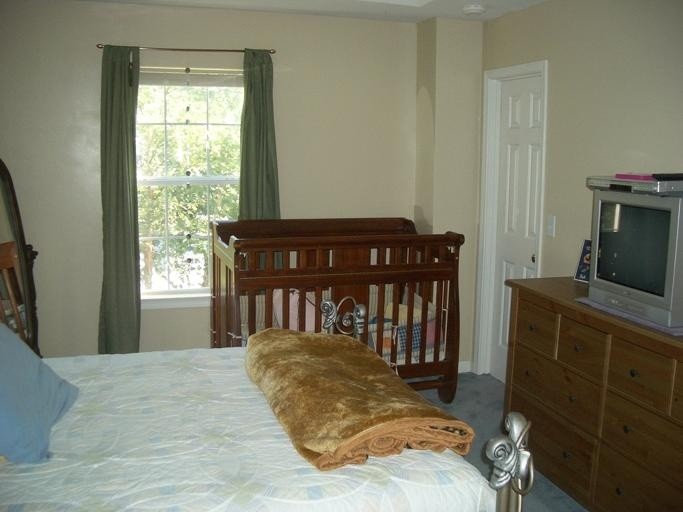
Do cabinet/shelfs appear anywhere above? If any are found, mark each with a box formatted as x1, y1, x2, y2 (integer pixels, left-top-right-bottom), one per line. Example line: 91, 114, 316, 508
503, 276, 683, 512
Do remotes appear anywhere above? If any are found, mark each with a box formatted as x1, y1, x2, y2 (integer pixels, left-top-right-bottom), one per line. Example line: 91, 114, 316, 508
652, 173, 683, 181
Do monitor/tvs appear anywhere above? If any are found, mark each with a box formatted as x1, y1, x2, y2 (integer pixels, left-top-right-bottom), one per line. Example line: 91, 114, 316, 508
588, 189, 683, 328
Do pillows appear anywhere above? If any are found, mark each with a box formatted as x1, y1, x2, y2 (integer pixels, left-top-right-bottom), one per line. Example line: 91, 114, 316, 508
0, 323, 79, 464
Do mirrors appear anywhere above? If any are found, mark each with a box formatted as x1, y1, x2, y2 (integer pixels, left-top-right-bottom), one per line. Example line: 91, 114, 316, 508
0, 158, 43, 358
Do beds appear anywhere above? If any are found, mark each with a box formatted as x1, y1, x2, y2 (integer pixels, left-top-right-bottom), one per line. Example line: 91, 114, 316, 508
208, 217, 464, 404
0, 347, 535, 511
0, 242, 30, 344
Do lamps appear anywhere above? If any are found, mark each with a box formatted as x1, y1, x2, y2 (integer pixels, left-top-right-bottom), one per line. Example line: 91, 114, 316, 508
462, 3, 486, 17
